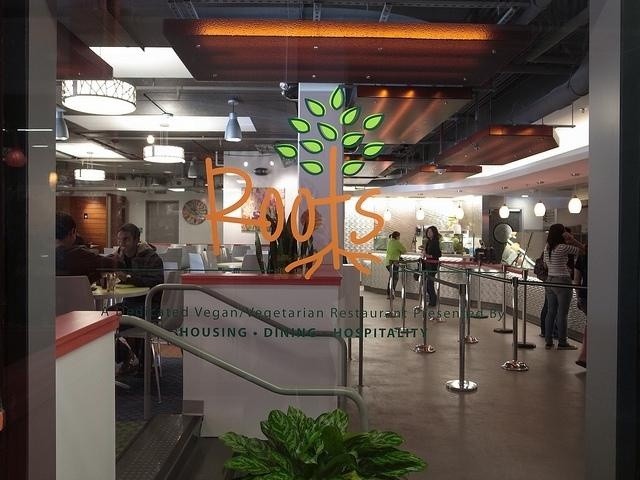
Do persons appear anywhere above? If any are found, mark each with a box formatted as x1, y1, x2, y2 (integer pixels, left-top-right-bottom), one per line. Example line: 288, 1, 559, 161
540, 227, 575, 340
385, 232, 406, 300
542, 223, 585, 350
417, 226, 442, 307
56, 212, 127, 287
106, 222, 164, 380
574, 243, 588, 369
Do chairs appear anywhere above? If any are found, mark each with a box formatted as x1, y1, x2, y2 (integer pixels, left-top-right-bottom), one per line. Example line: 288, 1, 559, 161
55, 243, 280, 404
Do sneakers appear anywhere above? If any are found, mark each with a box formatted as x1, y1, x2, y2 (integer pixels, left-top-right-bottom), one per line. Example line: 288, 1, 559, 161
136, 363, 144, 379
118, 350, 134, 374
545, 343, 553, 349
574, 358, 586, 370
557, 343, 578, 351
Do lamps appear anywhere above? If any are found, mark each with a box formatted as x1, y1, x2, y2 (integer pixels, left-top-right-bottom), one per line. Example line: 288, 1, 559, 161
224, 99, 243, 142
61, 75, 137, 116
142, 131, 186, 164
498, 186, 510, 219
416, 193, 424, 221
455, 189, 464, 219
383, 195, 392, 221
55, 106, 69, 141
74, 159, 106, 182
568, 172, 582, 214
534, 180, 547, 217
187, 161, 198, 178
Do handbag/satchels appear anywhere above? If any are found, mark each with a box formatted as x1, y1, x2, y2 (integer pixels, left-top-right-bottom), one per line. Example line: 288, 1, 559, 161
533, 250, 549, 282
414, 258, 427, 282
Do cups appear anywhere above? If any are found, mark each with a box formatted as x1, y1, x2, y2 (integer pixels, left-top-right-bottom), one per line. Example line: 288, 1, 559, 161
105, 272, 117, 293
